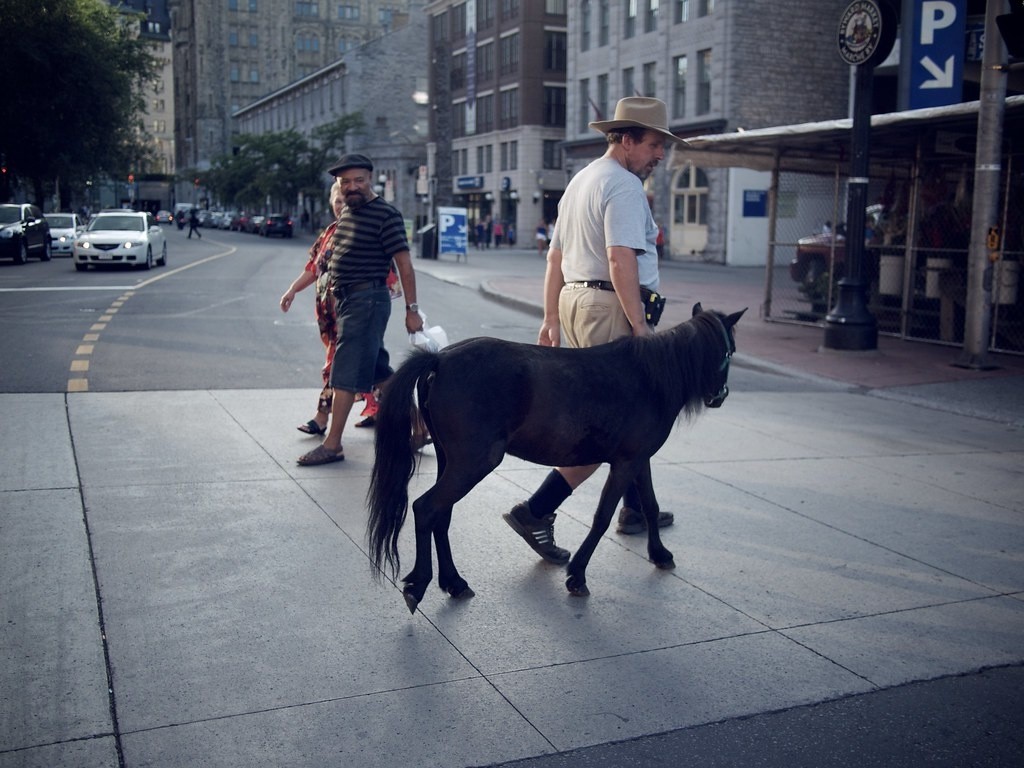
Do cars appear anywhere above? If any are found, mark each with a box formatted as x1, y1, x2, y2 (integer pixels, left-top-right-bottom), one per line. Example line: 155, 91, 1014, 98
41, 212, 87, 257
792, 202, 886, 298
171, 202, 265, 235
73, 209, 167, 271
155, 209, 173, 225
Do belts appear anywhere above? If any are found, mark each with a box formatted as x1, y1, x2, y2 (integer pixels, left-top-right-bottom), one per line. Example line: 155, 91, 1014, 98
579, 280, 652, 305
333, 278, 386, 297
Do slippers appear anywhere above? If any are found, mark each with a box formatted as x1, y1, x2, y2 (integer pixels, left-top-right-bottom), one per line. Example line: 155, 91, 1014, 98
296, 419, 327, 435
296, 443, 345, 466
410, 435, 432, 449
355, 415, 377, 427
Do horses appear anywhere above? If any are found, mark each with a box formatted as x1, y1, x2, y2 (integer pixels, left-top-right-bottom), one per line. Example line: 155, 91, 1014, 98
360, 301, 750, 618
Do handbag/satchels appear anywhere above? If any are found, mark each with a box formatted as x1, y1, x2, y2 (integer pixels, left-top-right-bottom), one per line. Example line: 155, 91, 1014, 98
408, 309, 448, 355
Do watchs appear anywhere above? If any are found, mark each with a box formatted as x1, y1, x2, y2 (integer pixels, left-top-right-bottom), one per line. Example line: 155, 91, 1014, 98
406, 303, 419, 312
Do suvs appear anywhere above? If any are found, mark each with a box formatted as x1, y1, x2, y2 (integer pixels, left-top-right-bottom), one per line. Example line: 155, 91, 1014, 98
0, 202, 53, 266
258, 212, 294, 235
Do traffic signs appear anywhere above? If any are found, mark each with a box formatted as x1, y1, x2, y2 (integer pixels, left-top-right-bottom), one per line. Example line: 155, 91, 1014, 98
437, 211, 469, 255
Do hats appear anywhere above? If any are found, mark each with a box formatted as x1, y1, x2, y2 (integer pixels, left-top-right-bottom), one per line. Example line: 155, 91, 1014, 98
328, 154, 373, 176
589, 97, 691, 146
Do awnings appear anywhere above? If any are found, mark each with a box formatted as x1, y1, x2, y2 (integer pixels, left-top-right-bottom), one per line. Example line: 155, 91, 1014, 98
666, 94, 1024, 318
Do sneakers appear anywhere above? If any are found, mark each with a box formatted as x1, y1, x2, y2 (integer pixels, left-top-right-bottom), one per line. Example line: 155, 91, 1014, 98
616, 508, 674, 534
502, 501, 571, 563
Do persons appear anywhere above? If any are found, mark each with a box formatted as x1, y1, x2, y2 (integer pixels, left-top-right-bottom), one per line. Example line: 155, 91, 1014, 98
279, 153, 433, 465
825, 215, 875, 246
187, 210, 202, 239
657, 223, 667, 257
468, 214, 556, 258
503, 96, 693, 563
